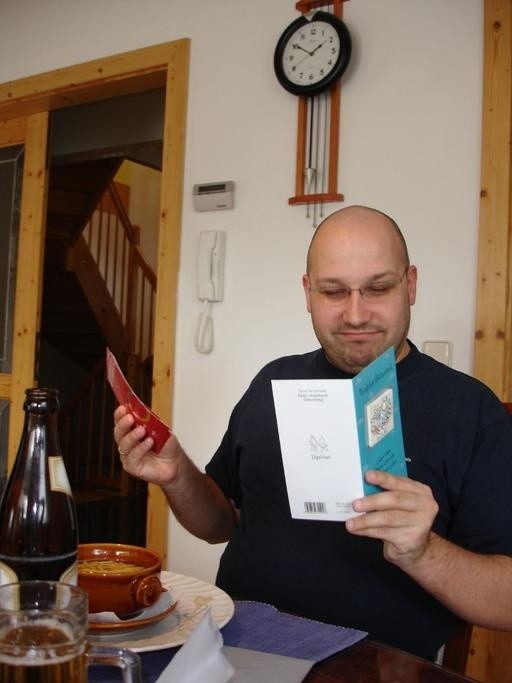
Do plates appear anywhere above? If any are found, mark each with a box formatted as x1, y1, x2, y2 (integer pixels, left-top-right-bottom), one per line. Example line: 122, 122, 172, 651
89, 570, 236, 653
83, 588, 175, 629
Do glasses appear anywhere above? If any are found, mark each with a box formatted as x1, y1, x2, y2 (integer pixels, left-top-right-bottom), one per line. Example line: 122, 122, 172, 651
310, 266, 409, 303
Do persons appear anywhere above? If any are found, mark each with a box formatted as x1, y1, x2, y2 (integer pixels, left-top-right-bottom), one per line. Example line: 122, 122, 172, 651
110, 203, 512, 666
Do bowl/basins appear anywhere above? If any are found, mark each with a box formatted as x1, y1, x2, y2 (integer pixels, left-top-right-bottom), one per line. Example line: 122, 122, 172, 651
78, 544, 161, 615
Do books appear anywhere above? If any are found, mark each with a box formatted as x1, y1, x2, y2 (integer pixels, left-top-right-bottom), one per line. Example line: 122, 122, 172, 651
270, 343, 409, 523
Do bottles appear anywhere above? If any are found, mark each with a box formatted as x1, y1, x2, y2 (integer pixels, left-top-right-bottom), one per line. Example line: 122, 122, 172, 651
0, 388, 78, 625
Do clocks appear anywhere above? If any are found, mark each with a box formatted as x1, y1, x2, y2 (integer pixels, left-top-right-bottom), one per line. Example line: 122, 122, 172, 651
273, 10, 353, 98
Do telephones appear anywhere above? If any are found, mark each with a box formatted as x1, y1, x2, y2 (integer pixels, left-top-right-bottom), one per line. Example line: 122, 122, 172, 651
196, 229, 227, 304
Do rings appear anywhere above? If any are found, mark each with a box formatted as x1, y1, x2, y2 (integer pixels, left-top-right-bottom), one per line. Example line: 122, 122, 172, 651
117, 445, 126, 456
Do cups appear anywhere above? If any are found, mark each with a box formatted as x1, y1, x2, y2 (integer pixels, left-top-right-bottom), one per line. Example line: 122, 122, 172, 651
0, 581, 142, 683
